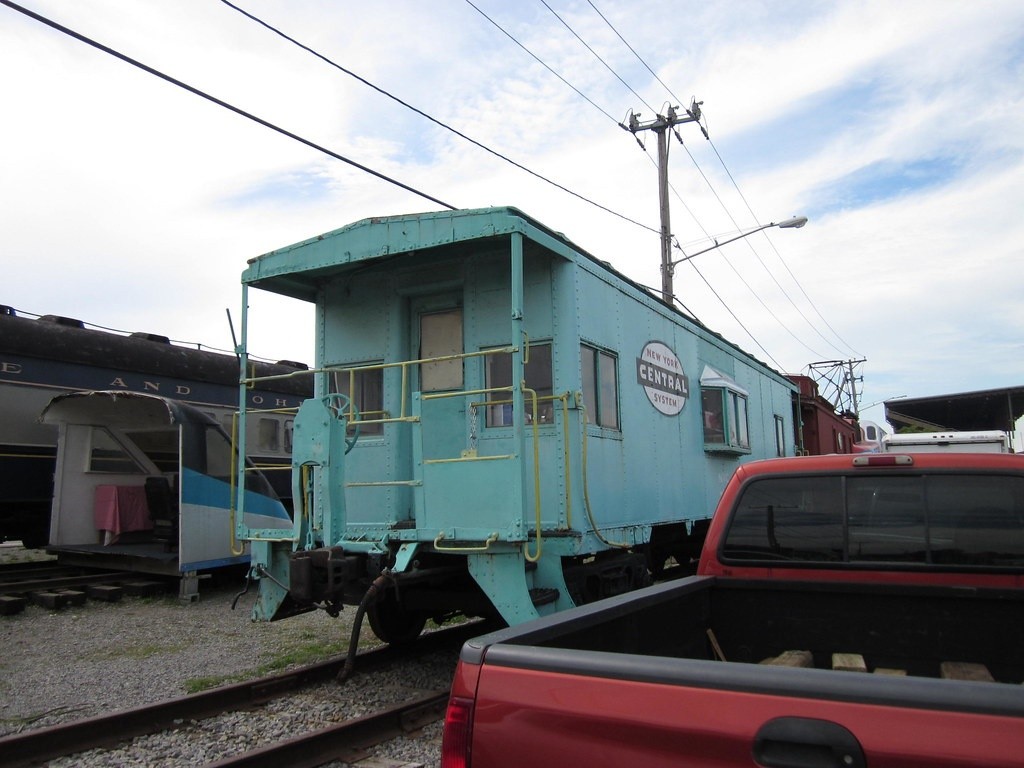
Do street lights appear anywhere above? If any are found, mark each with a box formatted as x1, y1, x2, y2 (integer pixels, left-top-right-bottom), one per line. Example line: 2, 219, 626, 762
662, 215, 808, 307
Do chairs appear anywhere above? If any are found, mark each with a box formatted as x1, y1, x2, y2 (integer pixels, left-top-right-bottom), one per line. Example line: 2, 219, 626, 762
144, 476, 178, 541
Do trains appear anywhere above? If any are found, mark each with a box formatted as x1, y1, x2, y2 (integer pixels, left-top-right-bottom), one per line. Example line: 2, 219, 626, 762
230, 205, 886, 683
1, 301, 314, 550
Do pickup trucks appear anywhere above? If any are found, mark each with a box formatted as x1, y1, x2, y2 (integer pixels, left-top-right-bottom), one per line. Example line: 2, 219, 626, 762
440, 451, 1024, 768
